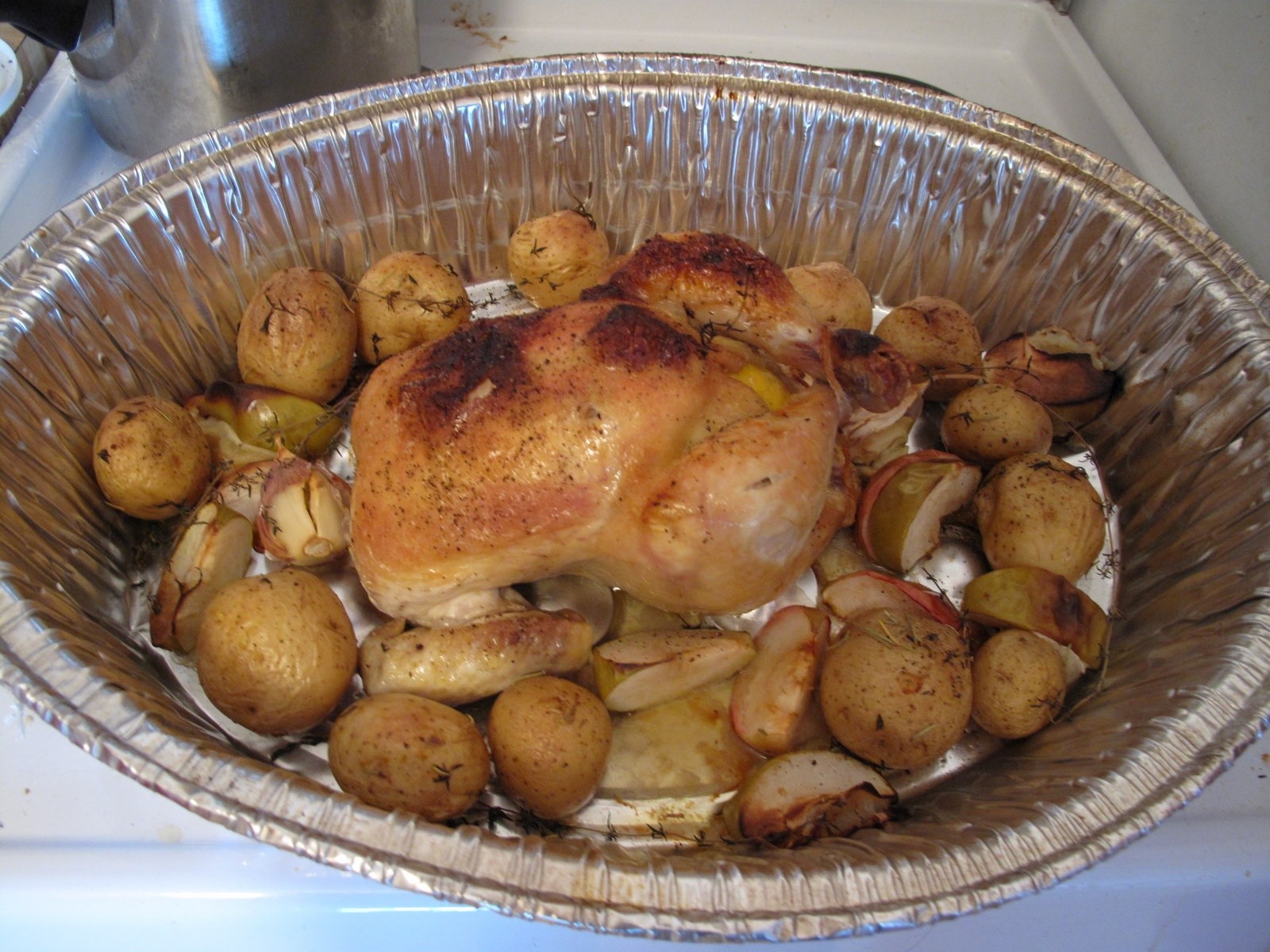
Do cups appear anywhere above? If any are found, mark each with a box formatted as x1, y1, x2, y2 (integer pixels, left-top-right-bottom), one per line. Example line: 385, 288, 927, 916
60, 0, 421, 159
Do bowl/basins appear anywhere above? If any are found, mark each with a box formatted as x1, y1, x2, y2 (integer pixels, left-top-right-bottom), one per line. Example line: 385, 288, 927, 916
0, 51, 1270, 942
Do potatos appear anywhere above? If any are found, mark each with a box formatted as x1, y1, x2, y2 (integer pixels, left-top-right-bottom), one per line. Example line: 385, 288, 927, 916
91, 211, 1107, 823
